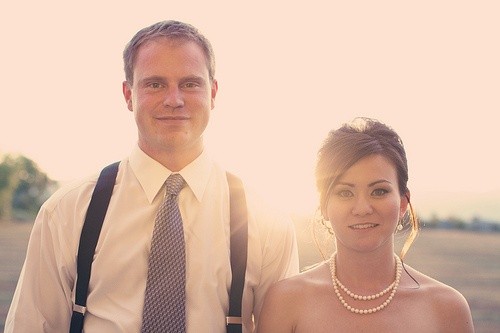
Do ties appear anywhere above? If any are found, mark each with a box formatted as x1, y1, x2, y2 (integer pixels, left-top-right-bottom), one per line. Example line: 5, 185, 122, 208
142, 175, 187, 333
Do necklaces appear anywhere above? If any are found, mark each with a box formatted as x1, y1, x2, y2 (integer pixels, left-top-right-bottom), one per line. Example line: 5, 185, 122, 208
330, 249, 403, 314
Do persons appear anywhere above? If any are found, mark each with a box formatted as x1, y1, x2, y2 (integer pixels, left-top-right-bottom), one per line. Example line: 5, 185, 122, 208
252, 116, 474, 333
4, 20, 301, 333
